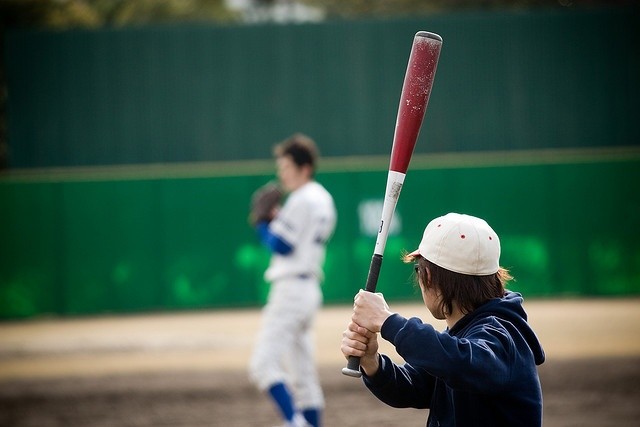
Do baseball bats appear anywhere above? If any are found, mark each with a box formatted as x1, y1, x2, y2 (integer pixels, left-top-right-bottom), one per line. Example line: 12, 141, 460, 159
342, 31, 442, 377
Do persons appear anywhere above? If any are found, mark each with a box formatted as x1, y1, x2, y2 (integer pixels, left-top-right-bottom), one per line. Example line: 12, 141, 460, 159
249, 134, 336, 427
340, 212, 547, 426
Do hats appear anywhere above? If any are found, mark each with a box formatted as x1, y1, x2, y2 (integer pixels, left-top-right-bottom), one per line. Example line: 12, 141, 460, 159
402, 212, 502, 277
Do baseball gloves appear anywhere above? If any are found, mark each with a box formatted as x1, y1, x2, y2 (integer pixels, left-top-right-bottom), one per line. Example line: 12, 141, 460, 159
251, 182, 283, 220
